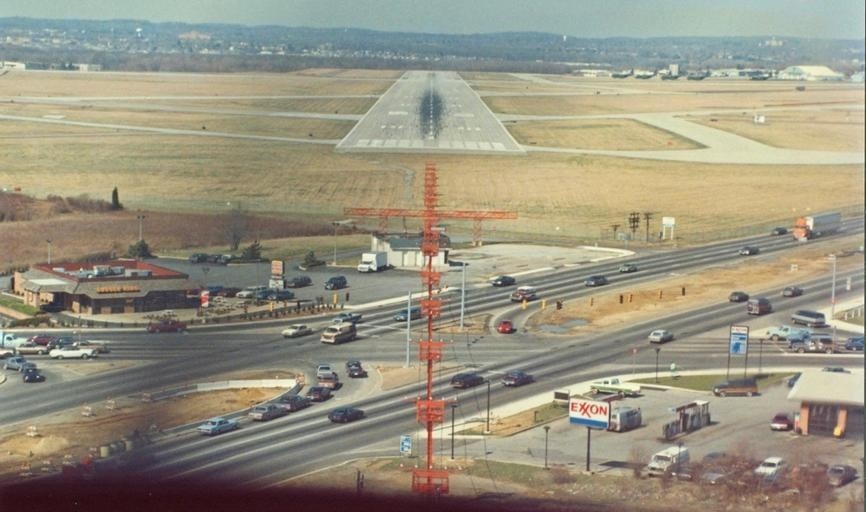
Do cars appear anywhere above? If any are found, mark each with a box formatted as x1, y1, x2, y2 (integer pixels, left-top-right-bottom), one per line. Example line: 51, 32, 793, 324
755, 457, 788, 477
822, 367, 851, 373
283, 323, 312, 338
767, 326, 865, 353
826, 463, 857, 486
741, 246, 760, 256
188, 275, 352, 301
190, 251, 236, 264
148, 320, 186, 334
499, 319, 514, 334
787, 371, 802, 386
772, 227, 788, 235
674, 451, 740, 487
64, 268, 101, 278
770, 412, 792, 431
494, 275, 515, 288
196, 359, 366, 437
504, 370, 535, 385
0, 334, 110, 383
649, 329, 675, 344
620, 263, 639, 272
585, 274, 606, 286
783, 286, 803, 297
730, 290, 749, 304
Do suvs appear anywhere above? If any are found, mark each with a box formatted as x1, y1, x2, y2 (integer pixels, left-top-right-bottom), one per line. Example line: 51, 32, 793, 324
749, 297, 773, 315
451, 372, 484, 388
512, 285, 537, 302
713, 378, 759, 398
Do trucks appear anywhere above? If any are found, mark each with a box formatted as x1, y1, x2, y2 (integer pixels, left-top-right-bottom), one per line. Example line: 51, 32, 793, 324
647, 445, 690, 479
793, 211, 841, 241
356, 251, 388, 274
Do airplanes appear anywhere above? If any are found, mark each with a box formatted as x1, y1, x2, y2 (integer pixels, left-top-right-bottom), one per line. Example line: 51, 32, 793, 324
660, 71, 685, 81
635, 69, 659, 79
752, 72, 774, 80
611, 69, 635, 80
686, 70, 712, 81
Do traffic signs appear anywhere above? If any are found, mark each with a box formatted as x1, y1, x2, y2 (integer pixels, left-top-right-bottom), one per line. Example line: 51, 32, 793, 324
785, 264, 798, 270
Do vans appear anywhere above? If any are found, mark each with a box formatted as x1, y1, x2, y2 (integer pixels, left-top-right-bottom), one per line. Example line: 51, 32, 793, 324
321, 321, 358, 345
610, 405, 643, 432
393, 306, 422, 322
790, 309, 828, 329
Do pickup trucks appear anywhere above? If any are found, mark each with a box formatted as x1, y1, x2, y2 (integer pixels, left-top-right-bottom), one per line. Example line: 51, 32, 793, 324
332, 310, 364, 324
592, 377, 643, 398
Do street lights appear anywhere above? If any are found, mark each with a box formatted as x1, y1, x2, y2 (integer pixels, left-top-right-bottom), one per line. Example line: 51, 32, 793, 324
46, 238, 53, 265
450, 402, 456, 459
759, 337, 765, 372
137, 215, 145, 242
543, 425, 550, 469
654, 347, 660, 384
332, 222, 340, 265
483, 378, 492, 434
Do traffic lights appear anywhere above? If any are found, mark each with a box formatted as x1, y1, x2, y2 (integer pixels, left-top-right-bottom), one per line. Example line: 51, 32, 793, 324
632, 346, 638, 354
